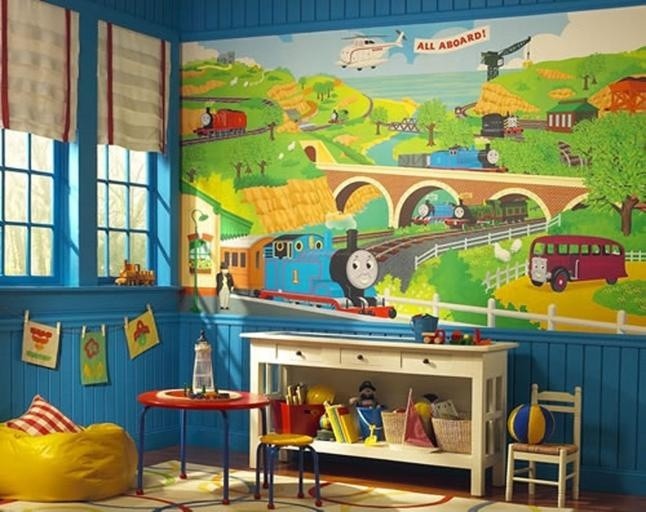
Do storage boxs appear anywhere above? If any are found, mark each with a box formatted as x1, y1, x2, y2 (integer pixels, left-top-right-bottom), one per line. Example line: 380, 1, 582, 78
271, 400, 325, 437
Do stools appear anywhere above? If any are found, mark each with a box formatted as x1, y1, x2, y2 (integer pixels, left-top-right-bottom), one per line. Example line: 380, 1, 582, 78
255, 433, 322, 509
0, 418, 138, 502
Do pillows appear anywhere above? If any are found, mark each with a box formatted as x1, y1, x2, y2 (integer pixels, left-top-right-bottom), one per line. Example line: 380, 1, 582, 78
7, 394, 84, 436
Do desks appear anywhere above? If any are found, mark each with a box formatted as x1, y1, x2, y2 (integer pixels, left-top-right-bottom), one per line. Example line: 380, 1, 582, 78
239, 330, 519, 497
136, 388, 273, 505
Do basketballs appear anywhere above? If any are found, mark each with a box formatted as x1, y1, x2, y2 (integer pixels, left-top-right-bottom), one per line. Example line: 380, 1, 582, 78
507, 404, 554, 444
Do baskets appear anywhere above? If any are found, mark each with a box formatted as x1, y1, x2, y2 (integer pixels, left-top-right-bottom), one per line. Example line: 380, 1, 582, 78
380, 408, 406, 445
431, 411, 472, 454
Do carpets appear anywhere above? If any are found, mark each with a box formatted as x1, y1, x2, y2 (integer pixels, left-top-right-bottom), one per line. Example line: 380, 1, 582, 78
0, 458, 572, 512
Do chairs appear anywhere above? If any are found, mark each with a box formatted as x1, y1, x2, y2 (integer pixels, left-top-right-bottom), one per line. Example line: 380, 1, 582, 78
505, 383, 582, 509
